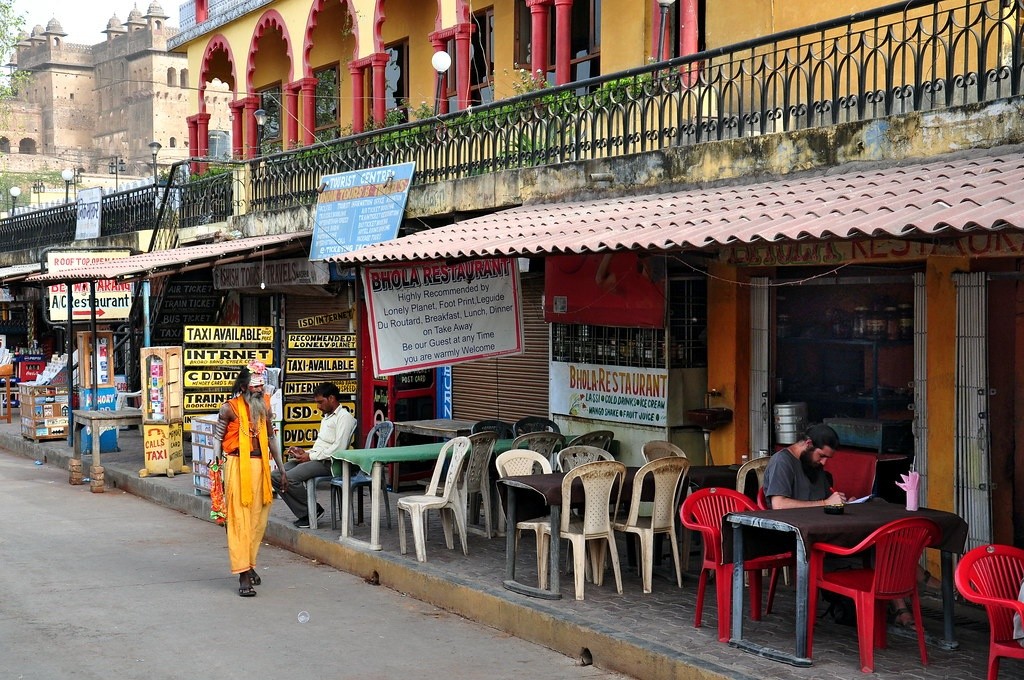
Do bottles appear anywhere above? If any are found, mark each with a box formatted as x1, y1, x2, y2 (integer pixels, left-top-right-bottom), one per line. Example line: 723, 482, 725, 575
741, 455, 747, 464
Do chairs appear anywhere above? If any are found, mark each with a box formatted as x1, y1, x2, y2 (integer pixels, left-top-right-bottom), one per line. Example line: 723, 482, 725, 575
679, 486, 793, 645
757, 486, 797, 616
562, 430, 614, 471
424, 431, 499, 549
396, 435, 471, 563
330, 420, 394, 530
557, 445, 615, 584
510, 431, 567, 474
300, 418, 358, 531
807, 516, 941, 673
471, 420, 514, 439
495, 449, 553, 591
513, 417, 560, 438
736, 456, 792, 588
641, 440, 693, 571
596, 456, 691, 595
540, 460, 628, 601
954, 544, 1024, 680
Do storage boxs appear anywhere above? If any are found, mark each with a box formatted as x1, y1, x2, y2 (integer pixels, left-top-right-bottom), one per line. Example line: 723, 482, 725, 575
17, 361, 45, 382
823, 416, 913, 456
15, 355, 45, 363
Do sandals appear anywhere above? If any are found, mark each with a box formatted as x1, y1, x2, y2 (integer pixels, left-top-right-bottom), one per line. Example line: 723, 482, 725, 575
891, 607, 925, 632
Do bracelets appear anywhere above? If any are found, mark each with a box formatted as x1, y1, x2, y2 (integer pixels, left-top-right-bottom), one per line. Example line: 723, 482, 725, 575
213, 418, 229, 442
268, 434, 276, 440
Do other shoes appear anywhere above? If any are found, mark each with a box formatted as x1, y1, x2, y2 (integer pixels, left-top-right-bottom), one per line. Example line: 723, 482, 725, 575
293, 502, 325, 527
239, 569, 262, 596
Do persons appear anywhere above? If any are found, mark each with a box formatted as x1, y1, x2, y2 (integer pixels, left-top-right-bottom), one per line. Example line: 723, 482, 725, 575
761, 422, 932, 636
269, 381, 358, 529
208, 361, 290, 597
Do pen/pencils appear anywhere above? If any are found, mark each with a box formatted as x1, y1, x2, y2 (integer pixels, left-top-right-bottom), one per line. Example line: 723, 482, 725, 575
285, 447, 302, 451
829, 487, 835, 493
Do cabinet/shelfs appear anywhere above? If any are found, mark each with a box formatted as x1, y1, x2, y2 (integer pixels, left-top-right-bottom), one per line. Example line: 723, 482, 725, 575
76, 330, 115, 389
140, 346, 184, 425
0, 364, 14, 424
775, 337, 913, 421
17, 381, 69, 444
190, 414, 218, 498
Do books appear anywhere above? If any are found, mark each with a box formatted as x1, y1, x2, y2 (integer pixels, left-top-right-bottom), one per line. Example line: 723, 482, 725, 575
79, 336, 109, 387
147, 356, 181, 422
18, 352, 73, 437
191, 413, 223, 491
0, 348, 12, 367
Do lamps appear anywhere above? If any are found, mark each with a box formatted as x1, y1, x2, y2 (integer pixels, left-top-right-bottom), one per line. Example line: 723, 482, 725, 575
108, 155, 126, 193
149, 142, 162, 183
62, 170, 74, 203
9, 186, 21, 216
657, 0, 675, 77
253, 109, 269, 158
431, 51, 451, 152
33, 179, 46, 210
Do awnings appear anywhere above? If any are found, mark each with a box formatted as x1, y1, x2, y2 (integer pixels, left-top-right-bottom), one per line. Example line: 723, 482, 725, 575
318, 146, 1024, 267
0, 228, 313, 288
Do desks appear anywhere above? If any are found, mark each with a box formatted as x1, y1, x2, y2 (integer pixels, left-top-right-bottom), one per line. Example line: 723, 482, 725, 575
393, 418, 480, 493
330, 438, 529, 553
721, 502, 970, 669
69, 409, 143, 466
495, 464, 759, 601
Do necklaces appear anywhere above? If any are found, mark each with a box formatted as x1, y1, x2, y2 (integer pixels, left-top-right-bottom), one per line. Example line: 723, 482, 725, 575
238, 398, 265, 438
